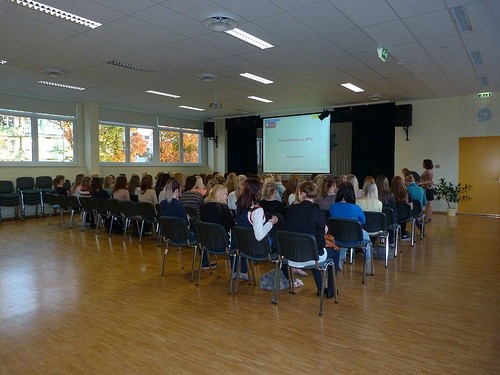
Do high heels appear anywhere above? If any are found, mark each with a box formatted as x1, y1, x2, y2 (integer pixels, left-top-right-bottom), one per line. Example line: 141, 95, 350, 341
425, 219, 432, 223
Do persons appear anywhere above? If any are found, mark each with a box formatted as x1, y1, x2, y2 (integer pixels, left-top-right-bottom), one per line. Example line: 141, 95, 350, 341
420, 159, 436, 223
284, 182, 339, 299
330, 182, 374, 275
53, 168, 427, 254
235, 178, 301, 288
199, 184, 253, 281
158, 179, 216, 269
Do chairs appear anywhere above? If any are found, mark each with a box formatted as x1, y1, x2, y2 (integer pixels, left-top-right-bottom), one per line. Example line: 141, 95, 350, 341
0, 176, 427, 316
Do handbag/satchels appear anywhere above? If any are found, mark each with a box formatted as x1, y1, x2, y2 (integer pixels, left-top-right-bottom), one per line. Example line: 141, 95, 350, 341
104, 218, 123, 234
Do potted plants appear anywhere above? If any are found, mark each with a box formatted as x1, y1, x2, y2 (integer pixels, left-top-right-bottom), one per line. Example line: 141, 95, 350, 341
433, 178, 474, 216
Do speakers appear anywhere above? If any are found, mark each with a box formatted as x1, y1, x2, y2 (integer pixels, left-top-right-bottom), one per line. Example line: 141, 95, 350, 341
395, 103, 412, 126
203, 122, 214, 138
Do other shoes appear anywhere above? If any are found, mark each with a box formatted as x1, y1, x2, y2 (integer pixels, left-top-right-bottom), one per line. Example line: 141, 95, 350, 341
202, 263, 217, 269
233, 272, 253, 280
318, 287, 327, 295
401, 235, 411, 240
86, 221, 91, 225
91, 224, 99, 229
326, 289, 338, 298
